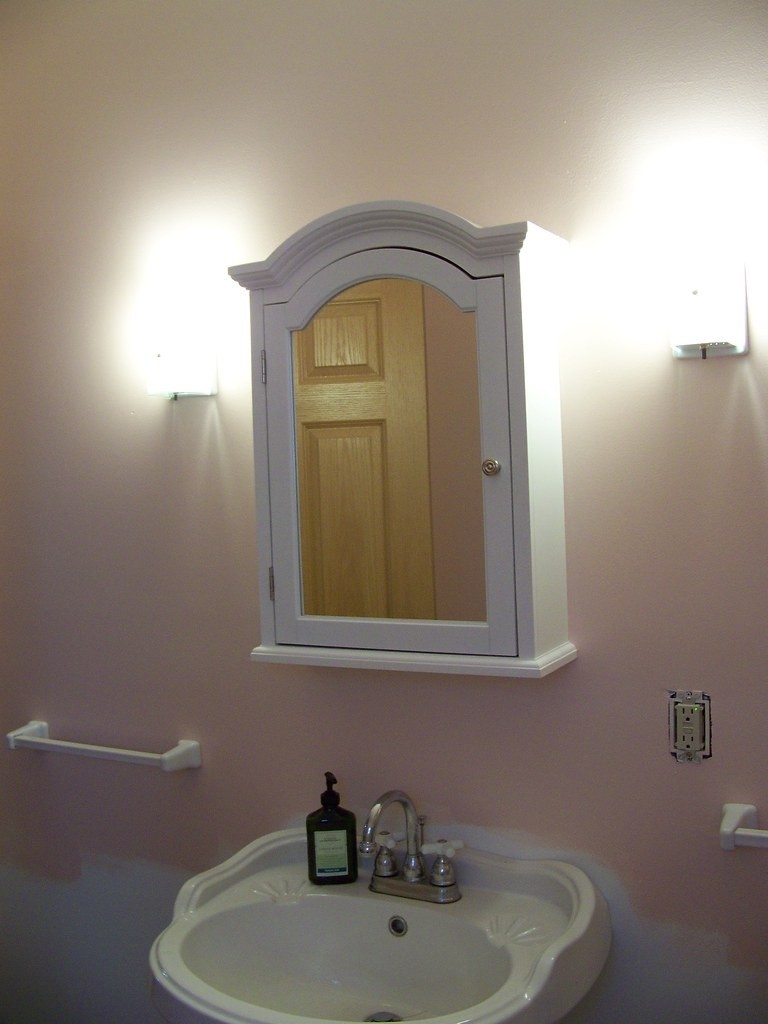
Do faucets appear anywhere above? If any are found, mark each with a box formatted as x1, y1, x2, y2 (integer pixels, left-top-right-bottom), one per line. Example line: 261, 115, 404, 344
358, 789, 427, 882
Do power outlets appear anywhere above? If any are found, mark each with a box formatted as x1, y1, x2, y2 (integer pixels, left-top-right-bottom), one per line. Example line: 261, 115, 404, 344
669, 691, 710, 764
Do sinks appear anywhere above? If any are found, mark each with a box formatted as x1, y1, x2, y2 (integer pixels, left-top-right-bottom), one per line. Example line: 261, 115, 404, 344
147, 823, 611, 1024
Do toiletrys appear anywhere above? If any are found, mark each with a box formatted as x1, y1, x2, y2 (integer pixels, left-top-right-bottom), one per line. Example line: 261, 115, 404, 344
305, 771, 357, 886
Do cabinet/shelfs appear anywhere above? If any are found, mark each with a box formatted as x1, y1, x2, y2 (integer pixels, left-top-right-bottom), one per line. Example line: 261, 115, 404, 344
229, 200, 576, 677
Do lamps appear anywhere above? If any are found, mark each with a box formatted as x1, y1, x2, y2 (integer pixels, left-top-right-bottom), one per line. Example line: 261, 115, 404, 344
667, 253, 749, 359
170, 334, 216, 399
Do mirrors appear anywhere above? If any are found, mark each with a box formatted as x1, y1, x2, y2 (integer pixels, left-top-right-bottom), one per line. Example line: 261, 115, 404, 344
264, 249, 517, 657
